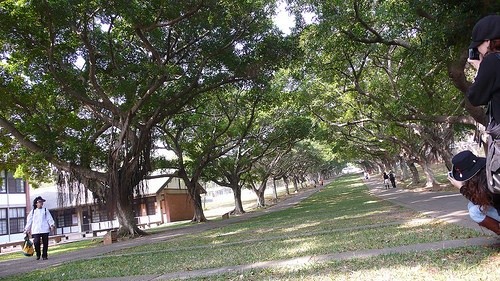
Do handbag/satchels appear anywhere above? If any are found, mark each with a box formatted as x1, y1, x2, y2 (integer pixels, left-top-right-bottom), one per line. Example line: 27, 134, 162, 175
485, 120, 500, 194
22, 232, 34, 256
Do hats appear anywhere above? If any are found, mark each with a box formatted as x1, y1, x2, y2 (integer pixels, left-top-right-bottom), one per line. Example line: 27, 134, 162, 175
448, 150, 486, 182
34, 197, 46, 203
467, 15, 500, 49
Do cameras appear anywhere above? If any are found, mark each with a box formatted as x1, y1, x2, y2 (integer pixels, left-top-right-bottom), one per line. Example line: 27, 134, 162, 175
449, 172, 453, 177
469, 49, 480, 60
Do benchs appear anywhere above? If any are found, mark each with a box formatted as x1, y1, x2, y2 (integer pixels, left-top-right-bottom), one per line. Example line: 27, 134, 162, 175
90, 221, 163, 236
0, 234, 66, 253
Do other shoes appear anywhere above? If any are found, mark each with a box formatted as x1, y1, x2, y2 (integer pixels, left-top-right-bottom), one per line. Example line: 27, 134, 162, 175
43, 257, 48, 260
36, 256, 40, 260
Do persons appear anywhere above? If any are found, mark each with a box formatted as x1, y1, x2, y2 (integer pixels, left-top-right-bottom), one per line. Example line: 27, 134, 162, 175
467, 15, 500, 193
383, 172, 390, 190
447, 150, 500, 235
24, 196, 55, 260
389, 172, 396, 188
